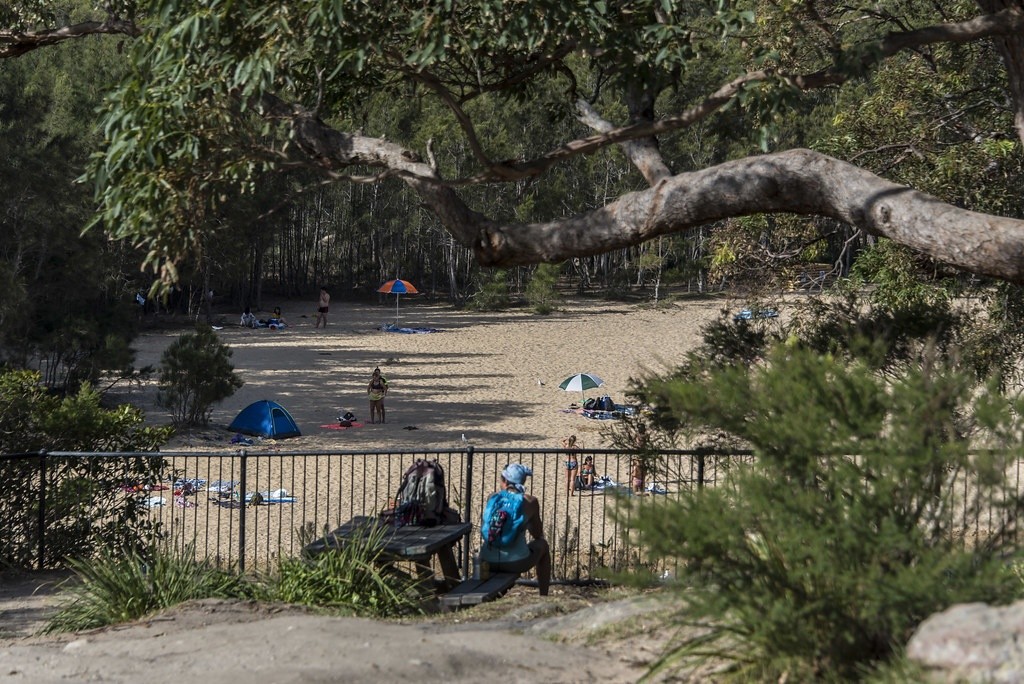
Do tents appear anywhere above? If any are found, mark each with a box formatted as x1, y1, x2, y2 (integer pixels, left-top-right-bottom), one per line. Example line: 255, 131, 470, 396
227, 398, 302, 440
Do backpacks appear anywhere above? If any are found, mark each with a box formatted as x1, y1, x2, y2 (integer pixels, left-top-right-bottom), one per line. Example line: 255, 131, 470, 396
394, 458, 447, 528
480, 489, 526, 547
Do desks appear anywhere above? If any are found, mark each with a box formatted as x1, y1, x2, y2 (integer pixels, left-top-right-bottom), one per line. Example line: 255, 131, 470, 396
300, 515, 472, 602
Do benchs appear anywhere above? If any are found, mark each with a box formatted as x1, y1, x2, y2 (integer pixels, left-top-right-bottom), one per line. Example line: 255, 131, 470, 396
732, 308, 780, 325
441, 573, 521, 612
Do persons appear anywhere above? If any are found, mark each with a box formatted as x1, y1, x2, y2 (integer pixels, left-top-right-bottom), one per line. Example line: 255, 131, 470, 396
314, 286, 330, 329
562, 434, 582, 497
241, 306, 257, 329
367, 365, 389, 424
478, 463, 551, 597
577, 455, 599, 490
272, 306, 293, 328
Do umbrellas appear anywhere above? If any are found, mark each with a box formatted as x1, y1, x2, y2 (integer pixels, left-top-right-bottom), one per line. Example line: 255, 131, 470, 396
736, 306, 778, 332
559, 372, 604, 406
376, 275, 420, 329
